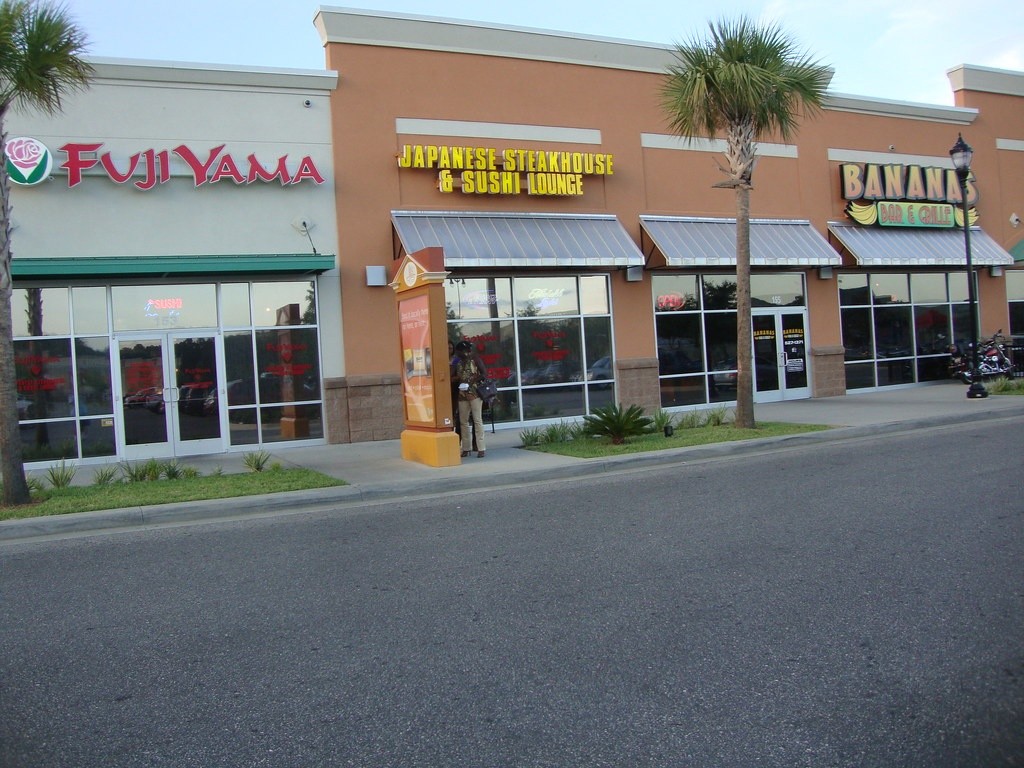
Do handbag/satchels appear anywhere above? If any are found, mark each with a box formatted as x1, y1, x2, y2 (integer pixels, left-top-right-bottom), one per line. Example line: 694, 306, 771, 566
476, 381, 498, 400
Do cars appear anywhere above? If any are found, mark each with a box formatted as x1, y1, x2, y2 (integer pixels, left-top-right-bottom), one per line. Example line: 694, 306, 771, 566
179, 373, 310, 417
843, 335, 944, 361
16, 390, 52, 419
497, 362, 568, 389
710, 357, 791, 391
569, 357, 614, 389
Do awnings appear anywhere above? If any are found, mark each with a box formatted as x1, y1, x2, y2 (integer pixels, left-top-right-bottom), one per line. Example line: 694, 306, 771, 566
639, 214, 843, 267
390, 210, 645, 266
826, 221, 1015, 266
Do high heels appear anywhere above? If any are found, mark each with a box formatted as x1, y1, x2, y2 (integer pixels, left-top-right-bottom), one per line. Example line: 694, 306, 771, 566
461, 451, 471, 456
478, 451, 485, 458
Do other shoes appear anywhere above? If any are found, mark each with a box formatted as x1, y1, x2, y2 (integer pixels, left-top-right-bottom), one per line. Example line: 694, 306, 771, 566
473, 442, 478, 451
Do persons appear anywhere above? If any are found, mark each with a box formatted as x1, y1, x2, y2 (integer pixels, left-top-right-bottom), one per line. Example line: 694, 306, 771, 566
68, 383, 90, 443
448, 339, 487, 458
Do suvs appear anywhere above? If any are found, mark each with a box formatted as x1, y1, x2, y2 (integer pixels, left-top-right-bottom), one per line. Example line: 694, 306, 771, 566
124, 386, 165, 413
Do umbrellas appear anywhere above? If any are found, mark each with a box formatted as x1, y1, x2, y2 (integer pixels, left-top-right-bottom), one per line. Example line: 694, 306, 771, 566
914, 310, 957, 350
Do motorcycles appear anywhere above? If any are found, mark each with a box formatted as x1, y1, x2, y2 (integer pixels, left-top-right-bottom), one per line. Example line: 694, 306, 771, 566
948, 328, 1015, 385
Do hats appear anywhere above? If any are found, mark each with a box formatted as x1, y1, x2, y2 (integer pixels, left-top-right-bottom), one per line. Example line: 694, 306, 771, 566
455, 341, 472, 351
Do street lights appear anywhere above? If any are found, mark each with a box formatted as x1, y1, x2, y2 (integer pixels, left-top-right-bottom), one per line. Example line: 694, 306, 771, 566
950, 131, 993, 400
